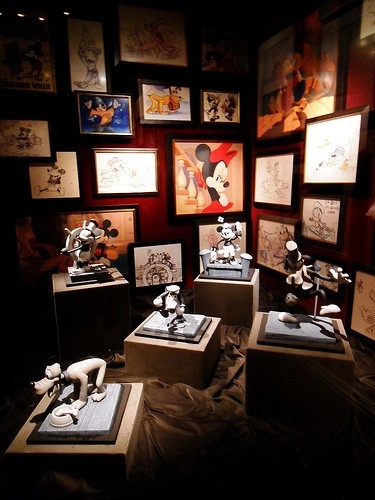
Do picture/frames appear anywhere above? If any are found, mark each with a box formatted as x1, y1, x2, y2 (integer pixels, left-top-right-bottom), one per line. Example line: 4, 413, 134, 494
309, 254, 349, 303
345, 261, 375, 352
128, 238, 186, 293
137, 78, 194, 124
192, 220, 251, 277
167, 133, 249, 226
299, 193, 345, 250
52, 205, 141, 269
0, 0, 161, 204
199, 88, 242, 126
253, 16, 371, 210
254, 215, 301, 278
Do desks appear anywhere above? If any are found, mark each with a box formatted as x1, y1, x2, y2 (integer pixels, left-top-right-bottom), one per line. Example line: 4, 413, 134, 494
243, 311, 355, 415
6, 382, 146, 500
124, 310, 225, 389
